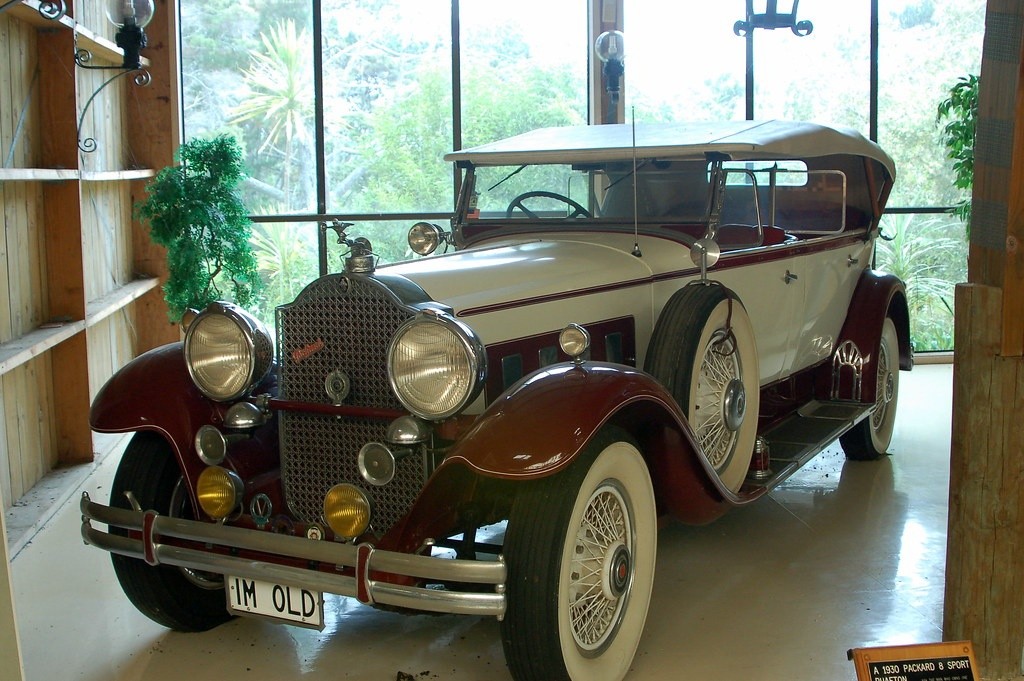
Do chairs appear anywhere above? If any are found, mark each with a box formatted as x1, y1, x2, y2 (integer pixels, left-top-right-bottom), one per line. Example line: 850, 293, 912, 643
766, 197, 870, 233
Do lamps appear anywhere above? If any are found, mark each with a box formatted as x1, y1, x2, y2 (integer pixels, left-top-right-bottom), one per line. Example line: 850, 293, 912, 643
73, 0, 156, 154
595, 30, 627, 125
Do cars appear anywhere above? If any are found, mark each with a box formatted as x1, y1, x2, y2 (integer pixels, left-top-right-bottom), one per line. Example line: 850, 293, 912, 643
79, 105, 914, 681
